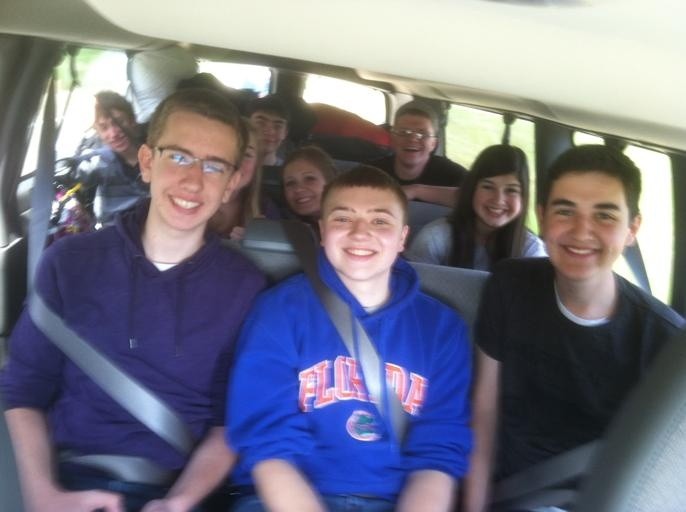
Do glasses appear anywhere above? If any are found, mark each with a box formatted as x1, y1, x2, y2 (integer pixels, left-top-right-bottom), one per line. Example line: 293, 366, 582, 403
150, 146, 238, 180
389, 127, 438, 142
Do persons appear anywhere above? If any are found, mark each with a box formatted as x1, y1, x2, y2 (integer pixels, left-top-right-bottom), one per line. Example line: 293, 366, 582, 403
0, 68, 686, 512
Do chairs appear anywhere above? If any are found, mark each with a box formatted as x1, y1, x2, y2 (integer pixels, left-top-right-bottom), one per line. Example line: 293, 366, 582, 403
545, 328, 686, 512
